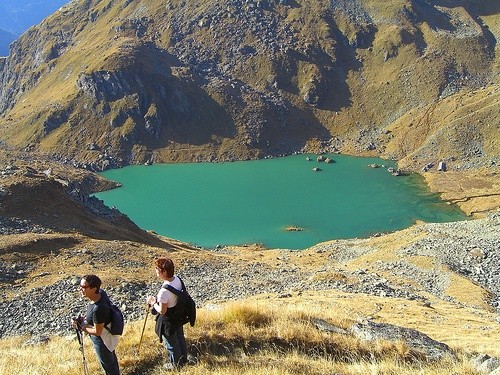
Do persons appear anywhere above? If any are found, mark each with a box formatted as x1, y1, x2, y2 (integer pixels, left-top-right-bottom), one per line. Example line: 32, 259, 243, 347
72, 274, 120, 375
147, 258, 187, 371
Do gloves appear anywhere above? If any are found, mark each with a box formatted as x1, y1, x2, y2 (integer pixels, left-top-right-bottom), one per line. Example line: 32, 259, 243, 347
147, 295, 156, 305
72, 313, 86, 331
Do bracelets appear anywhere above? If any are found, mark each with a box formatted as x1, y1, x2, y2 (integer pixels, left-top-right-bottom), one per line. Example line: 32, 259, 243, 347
153, 302, 156, 308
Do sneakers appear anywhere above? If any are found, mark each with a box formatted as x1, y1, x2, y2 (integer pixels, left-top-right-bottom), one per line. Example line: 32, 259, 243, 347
163, 363, 175, 371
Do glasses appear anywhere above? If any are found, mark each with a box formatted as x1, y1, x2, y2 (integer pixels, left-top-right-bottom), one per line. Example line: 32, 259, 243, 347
79, 284, 90, 289
156, 266, 162, 269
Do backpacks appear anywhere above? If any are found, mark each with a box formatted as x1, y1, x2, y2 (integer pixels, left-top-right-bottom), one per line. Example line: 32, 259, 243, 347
163, 276, 196, 327
92, 289, 124, 335
